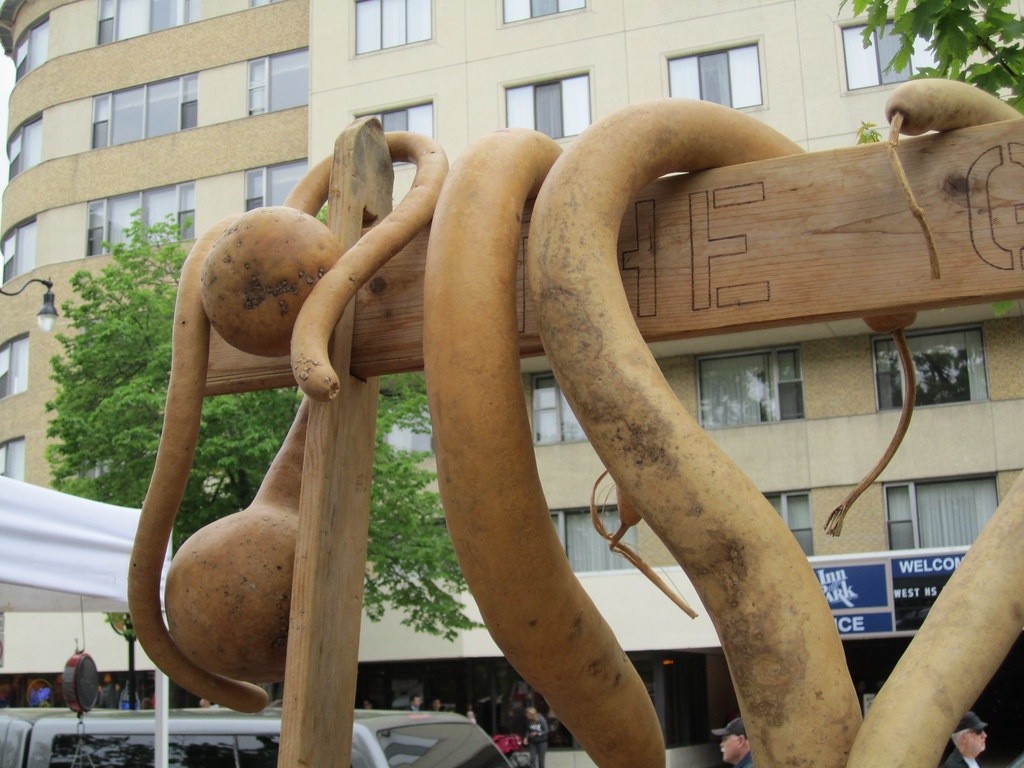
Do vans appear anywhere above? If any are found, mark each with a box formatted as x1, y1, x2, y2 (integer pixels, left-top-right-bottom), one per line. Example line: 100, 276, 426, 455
0, 696, 514, 768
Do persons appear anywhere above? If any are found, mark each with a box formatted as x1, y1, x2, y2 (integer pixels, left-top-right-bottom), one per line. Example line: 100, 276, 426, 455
200, 695, 1024, 768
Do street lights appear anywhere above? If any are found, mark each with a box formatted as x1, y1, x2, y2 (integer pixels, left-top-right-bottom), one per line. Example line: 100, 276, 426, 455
106, 609, 139, 711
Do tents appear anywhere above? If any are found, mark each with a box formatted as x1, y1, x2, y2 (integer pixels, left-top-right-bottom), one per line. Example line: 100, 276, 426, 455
0, 478, 172, 768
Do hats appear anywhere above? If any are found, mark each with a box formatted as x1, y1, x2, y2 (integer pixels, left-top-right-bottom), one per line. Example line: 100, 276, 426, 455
711, 717, 745, 736
954, 711, 988, 733
